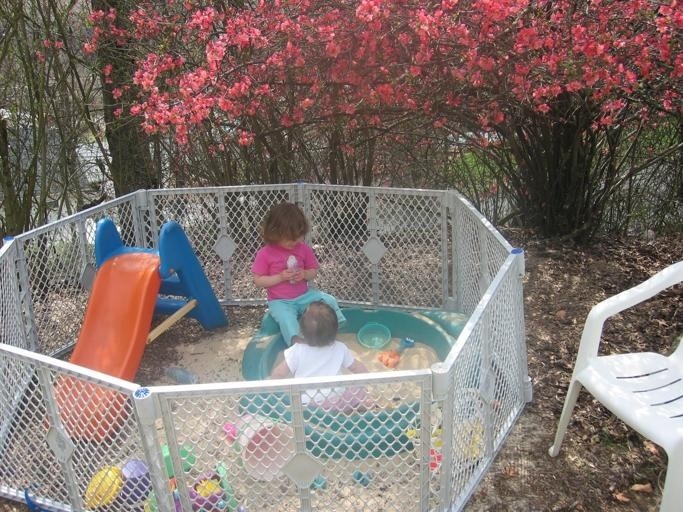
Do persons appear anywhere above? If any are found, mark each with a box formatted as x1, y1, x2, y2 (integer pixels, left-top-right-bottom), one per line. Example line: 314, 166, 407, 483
251, 202, 346, 348
265, 301, 377, 415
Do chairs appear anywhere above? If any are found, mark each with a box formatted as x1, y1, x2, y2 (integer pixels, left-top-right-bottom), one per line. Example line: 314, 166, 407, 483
548, 260, 683, 512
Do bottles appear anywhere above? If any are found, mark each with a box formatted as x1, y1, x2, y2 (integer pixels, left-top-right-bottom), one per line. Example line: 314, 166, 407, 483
167, 368, 199, 384
287, 255, 298, 282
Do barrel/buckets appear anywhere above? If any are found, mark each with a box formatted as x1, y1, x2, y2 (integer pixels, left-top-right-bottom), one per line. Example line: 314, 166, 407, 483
236, 415, 295, 482
